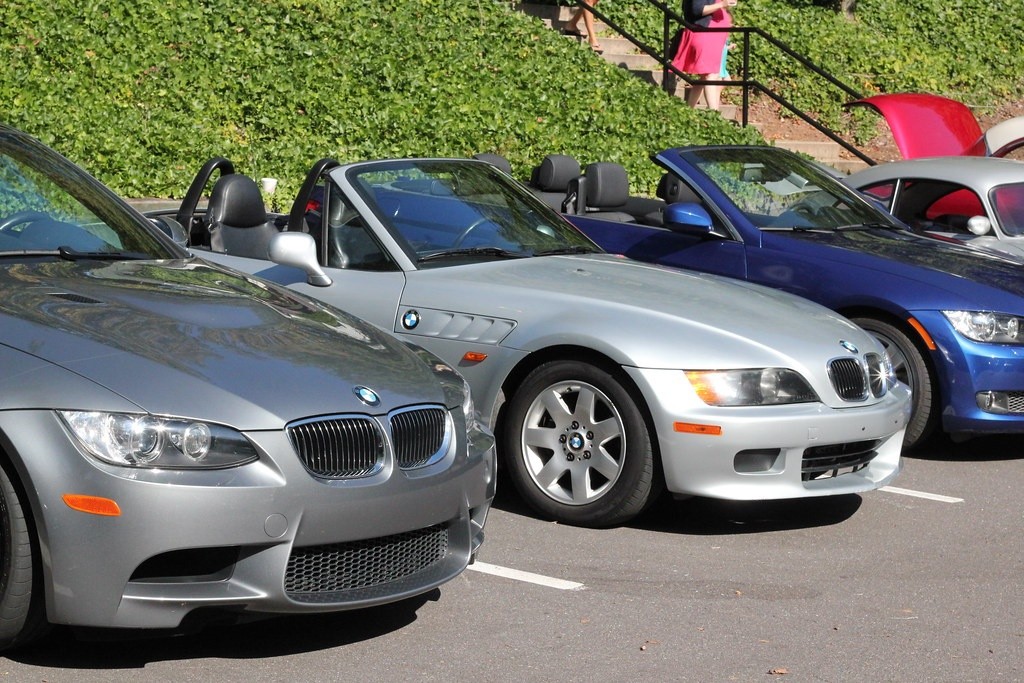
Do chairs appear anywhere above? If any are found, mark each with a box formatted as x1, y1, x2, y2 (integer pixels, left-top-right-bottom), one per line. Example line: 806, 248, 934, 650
666, 169, 729, 236
204, 174, 281, 261
330, 176, 388, 269
585, 161, 637, 226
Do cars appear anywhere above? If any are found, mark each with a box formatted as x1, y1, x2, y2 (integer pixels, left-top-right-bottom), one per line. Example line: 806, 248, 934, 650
844, 93, 1024, 226
784, 154, 1023, 265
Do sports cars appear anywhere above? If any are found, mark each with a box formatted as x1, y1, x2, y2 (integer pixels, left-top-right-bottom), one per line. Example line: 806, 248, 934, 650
0, 120, 499, 640
71, 157, 912, 529
367, 141, 1024, 448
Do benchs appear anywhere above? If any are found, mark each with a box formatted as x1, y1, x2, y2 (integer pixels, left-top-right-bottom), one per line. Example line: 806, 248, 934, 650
466, 154, 581, 213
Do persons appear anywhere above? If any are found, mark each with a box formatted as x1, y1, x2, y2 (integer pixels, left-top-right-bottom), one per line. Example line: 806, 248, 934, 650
668, 0, 737, 113
565, 0, 604, 55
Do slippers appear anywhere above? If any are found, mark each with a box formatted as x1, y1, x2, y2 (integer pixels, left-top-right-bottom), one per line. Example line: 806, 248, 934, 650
560, 27, 588, 38
587, 43, 606, 54
